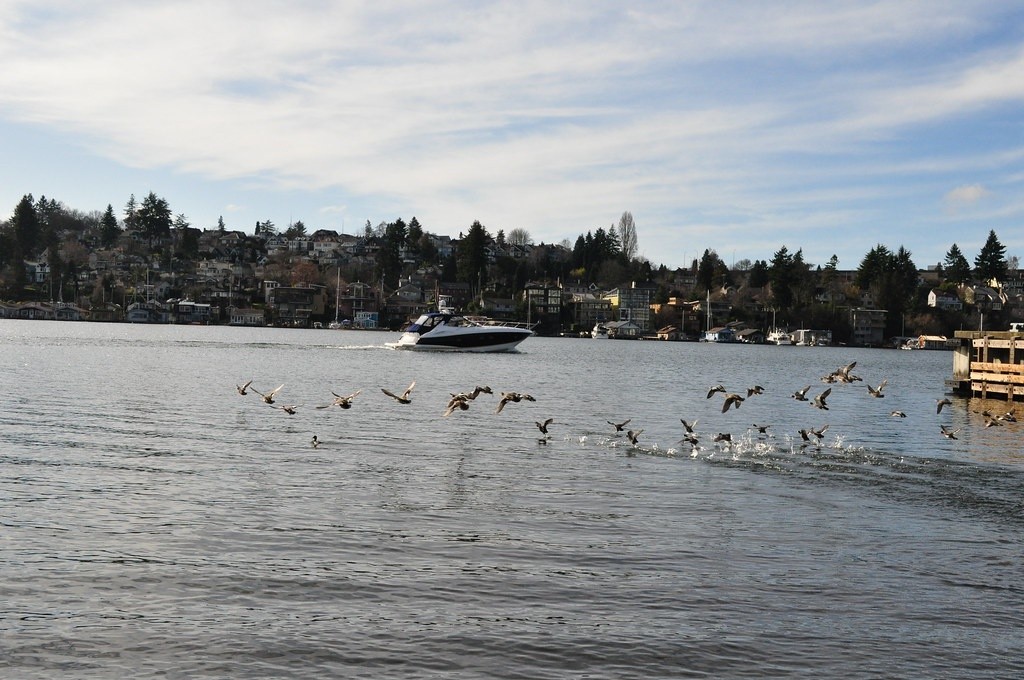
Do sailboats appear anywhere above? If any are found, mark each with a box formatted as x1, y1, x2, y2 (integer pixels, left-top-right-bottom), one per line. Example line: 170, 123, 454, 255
328, 267, 345, 330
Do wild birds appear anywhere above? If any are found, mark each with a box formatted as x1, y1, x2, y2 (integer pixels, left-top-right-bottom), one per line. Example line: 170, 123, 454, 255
235, 359, 1019, 446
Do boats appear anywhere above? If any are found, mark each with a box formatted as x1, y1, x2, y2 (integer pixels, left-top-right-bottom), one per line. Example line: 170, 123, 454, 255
384, 294, 542, 353
767, 310, 793, 345
